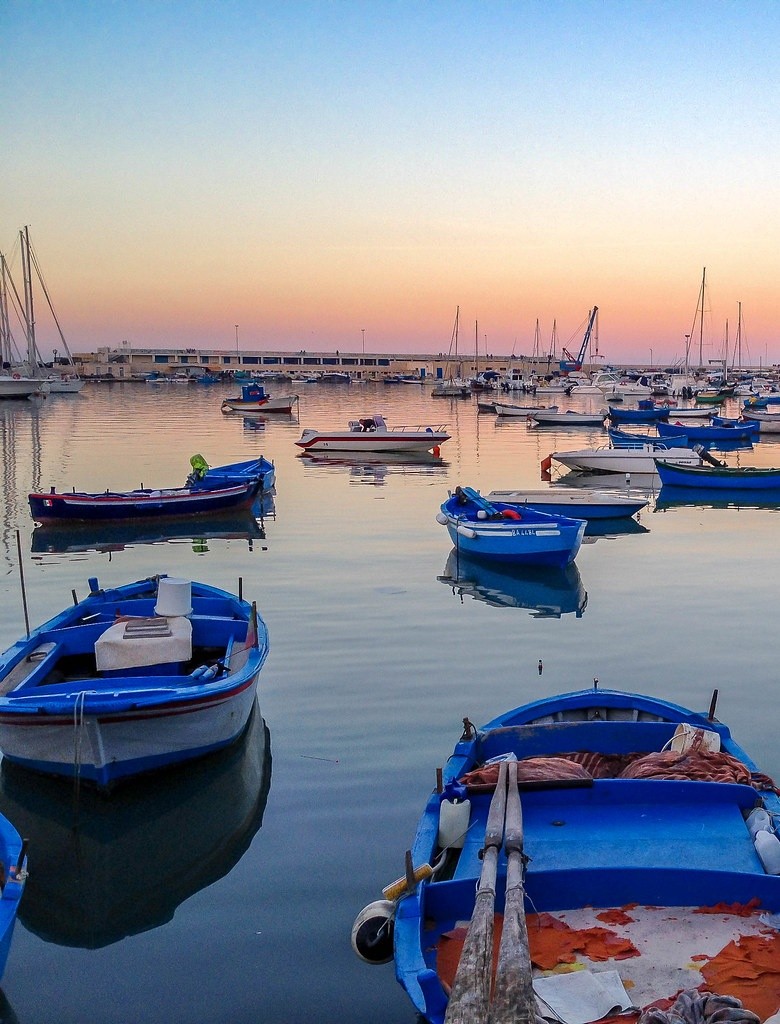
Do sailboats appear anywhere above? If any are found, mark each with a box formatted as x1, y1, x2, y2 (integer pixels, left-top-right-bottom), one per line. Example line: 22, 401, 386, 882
0, 226, 85, 398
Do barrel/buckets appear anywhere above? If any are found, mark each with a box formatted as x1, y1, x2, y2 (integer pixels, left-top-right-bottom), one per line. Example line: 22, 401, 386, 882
671, 723, 721, 754
154, 578, 193, 616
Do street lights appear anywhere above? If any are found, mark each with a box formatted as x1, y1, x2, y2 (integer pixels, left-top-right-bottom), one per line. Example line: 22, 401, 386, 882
360, 328, 365, 353
235, 324, 239, 364
685, 334, 690, 348
649, 348, 652, 364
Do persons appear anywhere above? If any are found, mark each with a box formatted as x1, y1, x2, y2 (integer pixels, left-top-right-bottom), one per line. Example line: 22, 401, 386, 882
360, 419, 377, 432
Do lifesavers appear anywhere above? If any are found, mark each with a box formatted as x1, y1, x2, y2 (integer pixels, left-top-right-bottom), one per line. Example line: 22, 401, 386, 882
501, 509, 523, 520
12, 372, 21, 379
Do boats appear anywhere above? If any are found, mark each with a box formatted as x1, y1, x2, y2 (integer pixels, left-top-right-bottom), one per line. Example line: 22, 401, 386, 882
351, 379, 367, 383
539, 442, 729, 475
293, 414, 453, 452
220, 382, 298, 413
347, 680, 780, 1024
29, 509, 266, 554
221, 409, 297, 430
1, 572, 269, 791
27, 472, 265, 526
293, 450, 443, 466
144, 368, 352, 384
248, 486, 277, 520
652, 483, 780, 513
653, 456, 780, 491
382, 266, 780, 448
183, 454, 276, 492
484, 489, 650, 519
436, 545, 588, 619
0, 694, 274, 950
435, 486, 588, 568
580, 518, 650, 544
370, 378, 379, 382
541, 469, 663, 490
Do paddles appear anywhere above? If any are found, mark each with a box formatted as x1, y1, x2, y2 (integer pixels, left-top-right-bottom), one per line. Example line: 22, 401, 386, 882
490, 761, 543, 1024
463, 485, 505, 519
443, 757, 507, 1024
